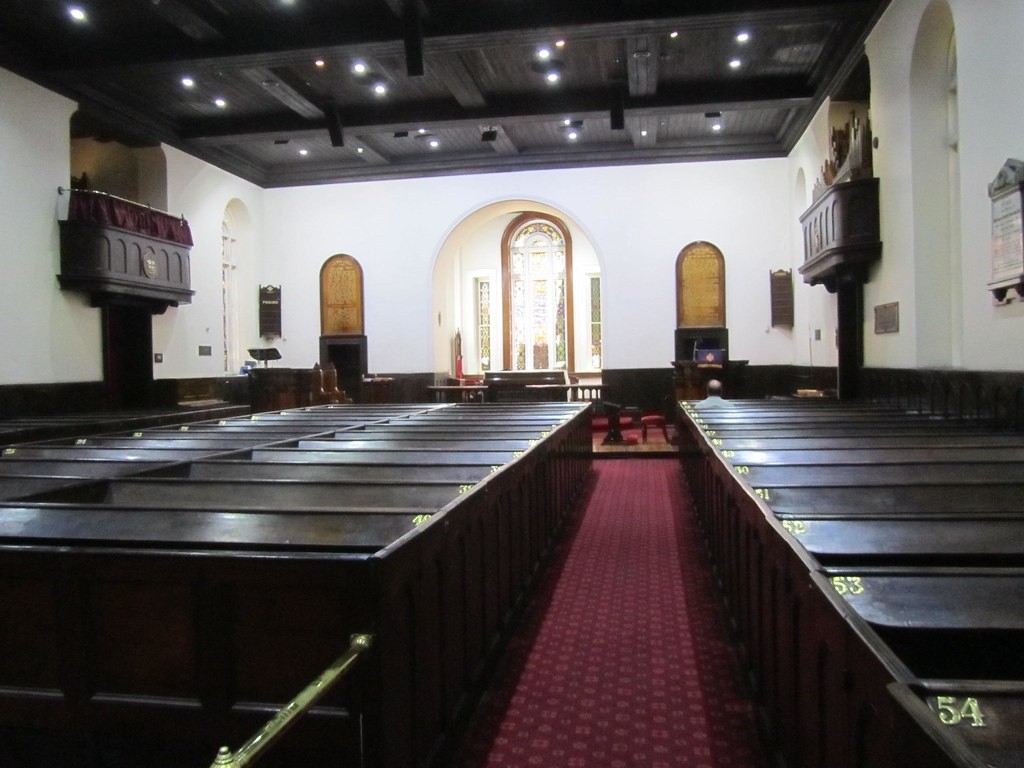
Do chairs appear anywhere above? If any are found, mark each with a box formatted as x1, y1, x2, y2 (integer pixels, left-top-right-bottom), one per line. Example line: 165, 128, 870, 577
312, 363, 335, 406
322, 362, 353, 403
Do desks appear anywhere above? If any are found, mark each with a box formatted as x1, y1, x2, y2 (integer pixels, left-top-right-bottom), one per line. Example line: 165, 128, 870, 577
482, 372, 568, 402
676, 398, 1023, 768
0, 403, 593, 768
361, 377, 396, 404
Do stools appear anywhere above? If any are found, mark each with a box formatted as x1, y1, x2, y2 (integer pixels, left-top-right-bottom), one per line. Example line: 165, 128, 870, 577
641, 415, 670, 444
